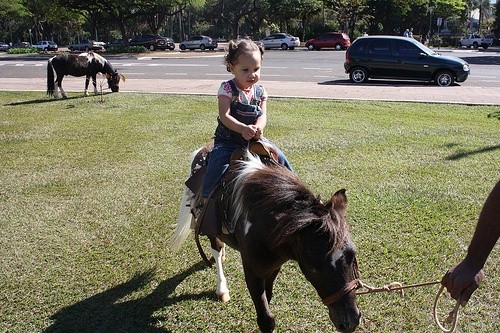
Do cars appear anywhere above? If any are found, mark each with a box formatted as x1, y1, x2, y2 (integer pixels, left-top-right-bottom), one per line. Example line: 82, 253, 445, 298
0, 42, 9, 52
343, 36, 470, 87
179, 36, 218, 51
305, 32, 350, 51
256, 33, 300, 51
68, 34, 175, 53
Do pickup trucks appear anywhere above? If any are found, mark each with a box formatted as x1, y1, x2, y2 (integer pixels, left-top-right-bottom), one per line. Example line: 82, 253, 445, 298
458, 34, 493, 50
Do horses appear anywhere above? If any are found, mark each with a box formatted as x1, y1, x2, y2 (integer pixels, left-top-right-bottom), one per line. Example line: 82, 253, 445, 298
45, 51, 122, 101
164, 141, 365, 333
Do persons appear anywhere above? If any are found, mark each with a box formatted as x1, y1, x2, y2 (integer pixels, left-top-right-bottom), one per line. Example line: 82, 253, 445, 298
441, 181, 500, 308
193, 40, 296, 220
404, 28, 432, 48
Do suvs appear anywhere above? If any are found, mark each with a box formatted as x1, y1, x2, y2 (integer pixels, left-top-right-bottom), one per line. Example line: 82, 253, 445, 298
30, 41, 58, 51
